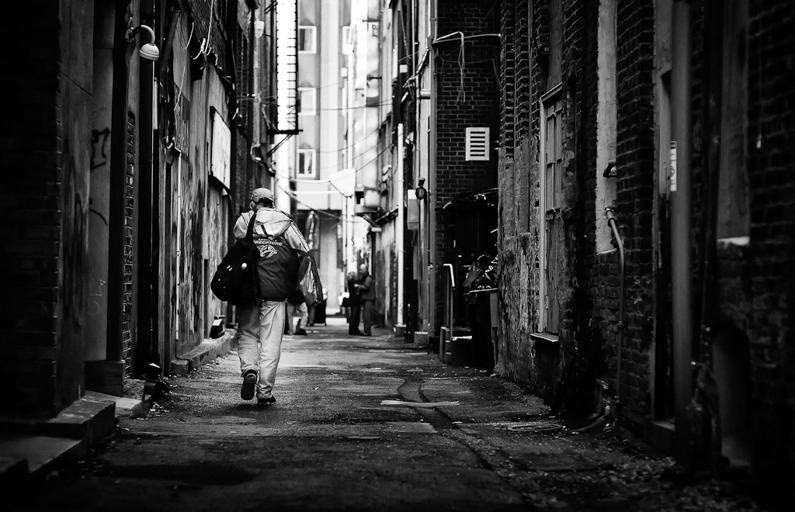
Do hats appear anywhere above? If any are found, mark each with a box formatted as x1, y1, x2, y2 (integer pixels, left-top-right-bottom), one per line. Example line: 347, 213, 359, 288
251, 188, 274, 202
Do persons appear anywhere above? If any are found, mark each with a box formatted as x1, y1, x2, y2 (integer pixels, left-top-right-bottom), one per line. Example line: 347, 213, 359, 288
307, 288, 317, 326
346, 271, 363, 336
286, 289, 308, 336
237, 189, 310, 405
354, 264, 375, 336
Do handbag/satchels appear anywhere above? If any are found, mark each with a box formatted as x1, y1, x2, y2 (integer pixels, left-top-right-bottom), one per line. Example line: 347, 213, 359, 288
258, 234, 309, 301
210, 239, 262, 308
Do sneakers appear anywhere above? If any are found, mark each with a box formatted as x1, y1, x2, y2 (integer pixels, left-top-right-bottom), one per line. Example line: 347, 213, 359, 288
241, 369, 276, 405
